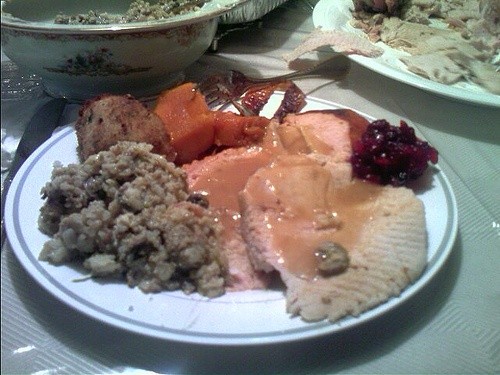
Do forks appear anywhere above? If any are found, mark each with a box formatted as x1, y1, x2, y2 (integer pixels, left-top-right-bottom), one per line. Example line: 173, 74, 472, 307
194, 54, 352, 110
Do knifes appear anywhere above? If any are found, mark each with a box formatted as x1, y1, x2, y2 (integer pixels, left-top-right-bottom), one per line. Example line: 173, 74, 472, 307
0, 95, 67, 261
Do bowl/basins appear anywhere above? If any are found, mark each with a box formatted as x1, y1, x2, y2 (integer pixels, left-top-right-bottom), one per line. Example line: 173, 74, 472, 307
0, 0, 253, 102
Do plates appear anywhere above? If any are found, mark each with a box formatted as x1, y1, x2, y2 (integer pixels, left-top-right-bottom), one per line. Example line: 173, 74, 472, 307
4, 86, 461, 347
311, 0, 500, 110
